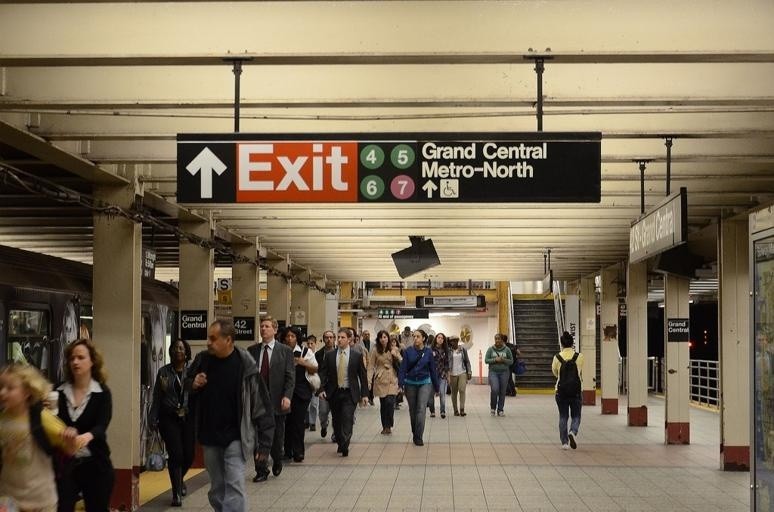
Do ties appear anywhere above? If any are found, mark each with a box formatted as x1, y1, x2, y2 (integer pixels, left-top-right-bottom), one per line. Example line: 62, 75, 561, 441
337, 352, 344, 386
260, 345, 269, 387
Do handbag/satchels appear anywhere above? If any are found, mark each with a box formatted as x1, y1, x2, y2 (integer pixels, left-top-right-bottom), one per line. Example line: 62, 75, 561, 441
146, 431, 165, 471
305, 368, 321, 391
513, 359, 526, 374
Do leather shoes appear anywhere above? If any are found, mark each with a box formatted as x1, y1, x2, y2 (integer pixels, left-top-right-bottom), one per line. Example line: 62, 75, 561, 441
272, 462, 282, 477
172, 479, 187, 507
253, 472, 268, 482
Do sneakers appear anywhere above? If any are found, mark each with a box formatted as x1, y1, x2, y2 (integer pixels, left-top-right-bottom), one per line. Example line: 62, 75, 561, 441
441, 413, 446, 418
561, 432, 578, 450
430, 413, 435, 417
285, 448, 304, 462
490, 409, 504, 417
454, 408, 466, 416
321, 428, 327, 437
414, 437, 423, 445
380, 428, 391, 434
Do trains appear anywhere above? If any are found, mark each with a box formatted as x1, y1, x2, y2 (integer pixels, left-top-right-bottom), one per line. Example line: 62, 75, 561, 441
0, 244, 178, 472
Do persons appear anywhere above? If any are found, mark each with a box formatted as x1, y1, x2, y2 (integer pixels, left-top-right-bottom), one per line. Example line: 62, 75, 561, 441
42, 336, 114, 512
551, 329, 585, 451
752, 325, 773, 478
279, 325, 369, 463
485, 334, 520, 417
248, 315, 296, 482
366, 326, 472, 434
0, 359, 83, 512
398, 330, 438, 446
146, 338, 197, 508
181, 318, 275, 512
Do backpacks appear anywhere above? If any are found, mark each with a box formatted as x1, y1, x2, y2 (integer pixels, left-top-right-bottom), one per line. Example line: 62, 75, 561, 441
555, 352, 580, 396
